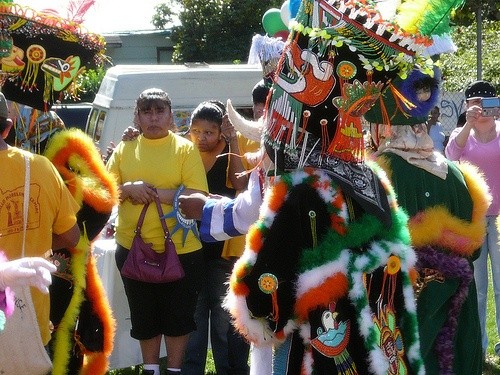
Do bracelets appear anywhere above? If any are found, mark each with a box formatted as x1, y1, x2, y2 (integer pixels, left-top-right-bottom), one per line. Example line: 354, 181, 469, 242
225, 136, 237, 142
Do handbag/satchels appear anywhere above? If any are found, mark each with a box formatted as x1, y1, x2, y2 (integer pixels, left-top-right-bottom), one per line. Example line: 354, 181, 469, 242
0, 286, 53, 375
121, 188, 184, 282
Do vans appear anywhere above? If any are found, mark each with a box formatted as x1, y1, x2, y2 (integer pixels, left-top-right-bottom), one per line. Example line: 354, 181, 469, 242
85, 62, 266, 164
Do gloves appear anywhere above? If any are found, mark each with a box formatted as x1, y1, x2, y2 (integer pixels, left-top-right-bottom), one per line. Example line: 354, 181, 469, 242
0, 256, 57, 294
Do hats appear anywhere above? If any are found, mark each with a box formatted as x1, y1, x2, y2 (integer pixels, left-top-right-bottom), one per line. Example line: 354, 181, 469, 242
464, 81, 497, 100
259, 0, 431, 174
0, 92, 9, 119
361, 0, 468, 128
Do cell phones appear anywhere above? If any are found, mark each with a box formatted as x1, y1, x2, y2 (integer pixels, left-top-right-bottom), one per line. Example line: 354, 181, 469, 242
480, 97, 500, 117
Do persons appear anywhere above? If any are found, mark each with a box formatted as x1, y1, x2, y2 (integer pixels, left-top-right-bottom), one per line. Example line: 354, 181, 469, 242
0, 0, 492, 375
445, 81, 500, 370
